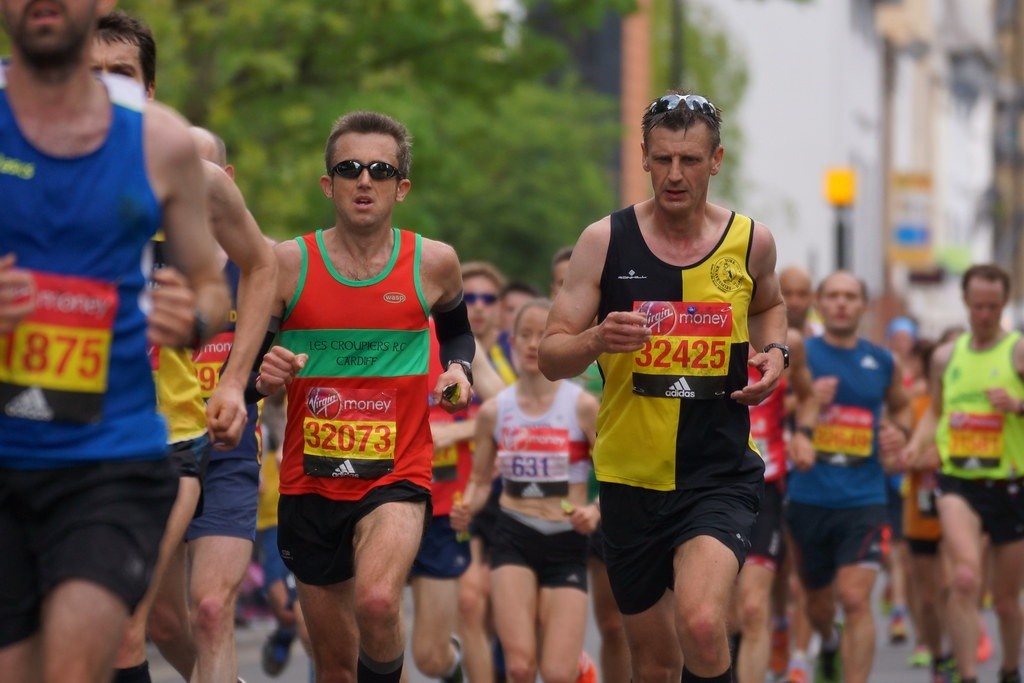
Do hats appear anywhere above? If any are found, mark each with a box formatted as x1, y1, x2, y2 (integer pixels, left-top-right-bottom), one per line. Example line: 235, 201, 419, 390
886, 314, 914, 338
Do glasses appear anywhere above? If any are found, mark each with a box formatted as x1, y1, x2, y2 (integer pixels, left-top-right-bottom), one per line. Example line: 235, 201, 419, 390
463, 293, 497, 306
330, 160, 403, 180
648, 94, 716, 115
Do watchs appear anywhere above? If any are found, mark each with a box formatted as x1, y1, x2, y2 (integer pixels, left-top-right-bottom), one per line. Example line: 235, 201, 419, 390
763, 342, 790, 369
194, 311, 206, 350
446, 358, 473, 386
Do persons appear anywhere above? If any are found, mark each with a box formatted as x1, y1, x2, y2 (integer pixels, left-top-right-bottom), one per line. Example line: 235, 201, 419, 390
747, 265, 1024, 683
90, 13, 317, 683
538, 95, 788, 683
219, 112, 476, 683
406, 246, 634, 682
0, 0, 231, 683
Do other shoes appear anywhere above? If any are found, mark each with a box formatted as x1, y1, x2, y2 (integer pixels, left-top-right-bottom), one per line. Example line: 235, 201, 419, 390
909, 637, 933, 667
441, 635, 465, 683
262, 618, 296, 676
785, 656, 812, 683
578, 651, 597, 683
976, 632, 992, 662
889, 610, 906, 644
930, 655, 960, 683
813, 623, 845, 683
770, 623, 789, 675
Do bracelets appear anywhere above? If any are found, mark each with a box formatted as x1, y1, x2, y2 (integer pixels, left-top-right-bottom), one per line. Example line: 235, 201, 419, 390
796, 425, 813, 437
